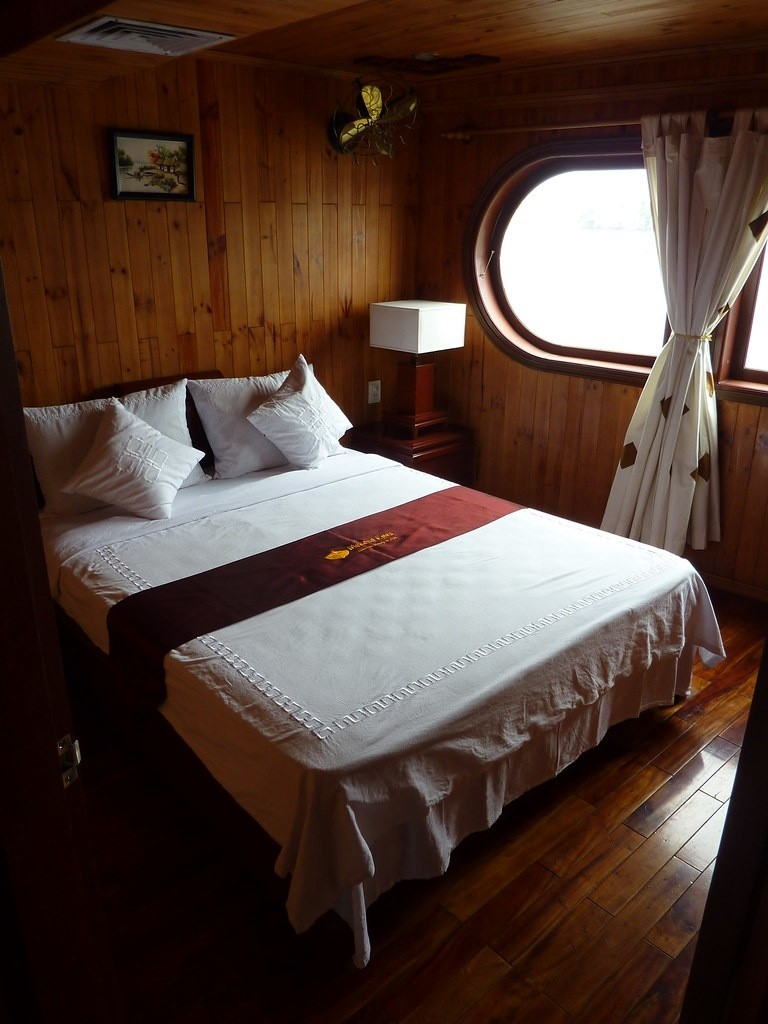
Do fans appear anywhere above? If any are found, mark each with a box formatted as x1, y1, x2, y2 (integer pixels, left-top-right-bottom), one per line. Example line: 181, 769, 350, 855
327, 70, 417, 168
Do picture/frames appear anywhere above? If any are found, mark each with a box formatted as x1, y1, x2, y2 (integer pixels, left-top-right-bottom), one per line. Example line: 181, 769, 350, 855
106, 128, 196, 202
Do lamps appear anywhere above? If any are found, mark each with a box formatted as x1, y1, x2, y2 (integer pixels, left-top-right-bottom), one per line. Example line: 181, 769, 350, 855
369, 299, 467, 439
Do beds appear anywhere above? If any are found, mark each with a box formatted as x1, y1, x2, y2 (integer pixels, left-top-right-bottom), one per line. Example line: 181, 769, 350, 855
22, 369, 726, 970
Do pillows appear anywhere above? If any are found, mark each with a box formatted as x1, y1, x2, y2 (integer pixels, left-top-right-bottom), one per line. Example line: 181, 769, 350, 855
186, 364, 321, 480
59, 396, 206, 520
23, 378, 212, 519
247, 353, 354, 467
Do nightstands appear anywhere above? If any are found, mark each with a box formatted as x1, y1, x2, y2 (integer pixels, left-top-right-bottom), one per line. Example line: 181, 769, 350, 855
352, 425, 473, 480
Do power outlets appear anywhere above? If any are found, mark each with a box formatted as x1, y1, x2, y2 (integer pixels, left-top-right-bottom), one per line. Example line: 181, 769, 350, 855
367, 380, 381, 404
